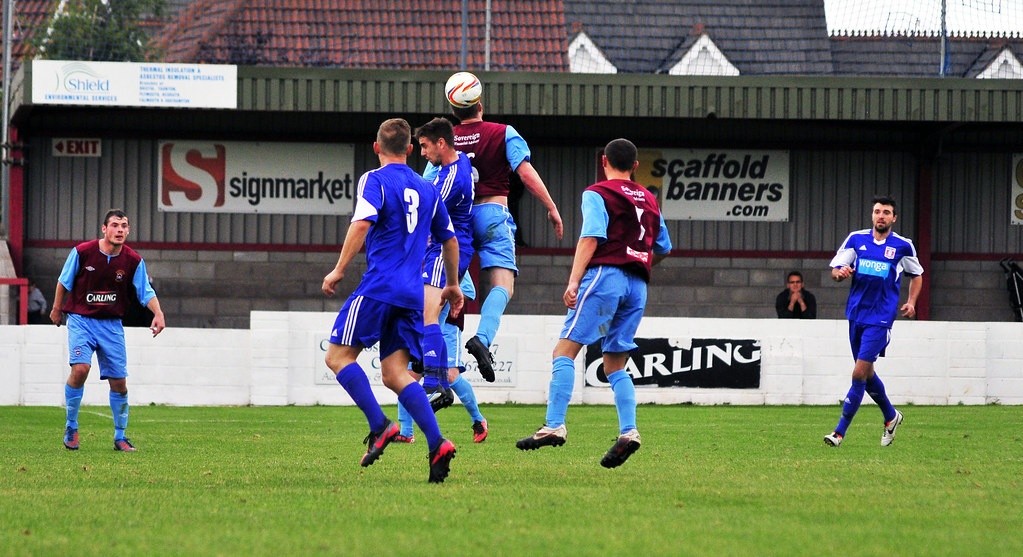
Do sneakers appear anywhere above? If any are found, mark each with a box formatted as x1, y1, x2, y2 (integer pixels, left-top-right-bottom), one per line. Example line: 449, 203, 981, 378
114, 440, 138, 453
360, 418, 400, 467
465, 336, 495, 382
424, 383, 454, 413
64, 426, 79, 450
880, 409, 903, 447
516, 424, 567, 450
396, 435, 415, 443
600, 429, 641, 469
428, 436, 456, 483
824, 432, 843, 447
472, 419, 488, 443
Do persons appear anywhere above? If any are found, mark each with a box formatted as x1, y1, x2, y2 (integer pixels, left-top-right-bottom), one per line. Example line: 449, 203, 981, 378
28, 280, 48, 325
823, 197, 924, 447
776, 271, 817, 319
422, 100, 563, 383
49, 210, 166, 452
402, 117, 475, 413
321, 118, 464, 483
516, 138, 672, 469
387, 269, 488, 442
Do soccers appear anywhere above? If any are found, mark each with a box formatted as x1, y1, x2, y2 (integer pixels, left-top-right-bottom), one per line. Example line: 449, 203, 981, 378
444, 71, 483, 109
